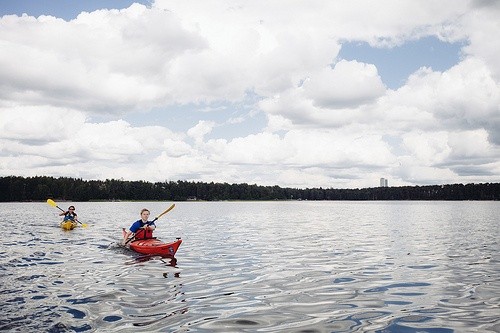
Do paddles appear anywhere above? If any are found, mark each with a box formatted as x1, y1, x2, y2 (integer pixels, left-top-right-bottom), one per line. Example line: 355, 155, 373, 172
125, 203, 175, 244
47, 199, 88, 228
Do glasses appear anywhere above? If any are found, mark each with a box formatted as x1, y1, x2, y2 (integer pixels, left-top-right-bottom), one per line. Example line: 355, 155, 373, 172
69, 208, 75, 210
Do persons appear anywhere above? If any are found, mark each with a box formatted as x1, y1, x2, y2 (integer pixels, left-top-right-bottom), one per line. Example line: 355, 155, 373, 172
122, 209, 156, 245
59, 206, 77, 220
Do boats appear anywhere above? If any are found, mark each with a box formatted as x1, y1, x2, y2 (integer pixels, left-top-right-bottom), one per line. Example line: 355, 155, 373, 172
60, 218, 77, 230
122, 227, 182, 256
